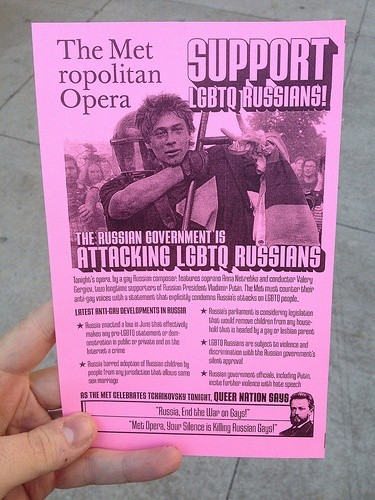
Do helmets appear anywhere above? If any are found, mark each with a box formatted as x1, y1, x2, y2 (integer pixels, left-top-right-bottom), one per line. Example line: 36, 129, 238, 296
109, 111, 145, 146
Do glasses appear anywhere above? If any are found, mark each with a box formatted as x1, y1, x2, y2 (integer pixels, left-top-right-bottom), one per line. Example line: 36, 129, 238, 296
304, 165, 316, 170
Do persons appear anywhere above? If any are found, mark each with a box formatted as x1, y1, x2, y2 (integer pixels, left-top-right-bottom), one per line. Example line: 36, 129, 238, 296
63, 93, 326, 258
278, 391, 317, 437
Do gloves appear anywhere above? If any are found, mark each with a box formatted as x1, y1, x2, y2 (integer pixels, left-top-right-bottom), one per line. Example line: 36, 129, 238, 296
181, 149, 210, 181
